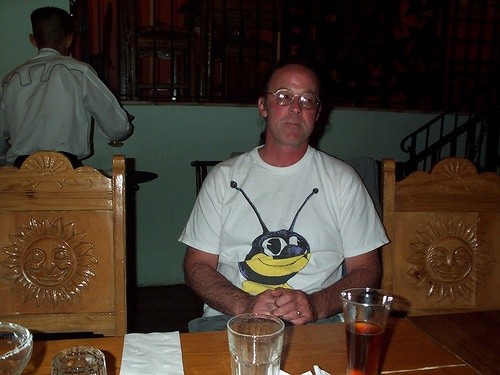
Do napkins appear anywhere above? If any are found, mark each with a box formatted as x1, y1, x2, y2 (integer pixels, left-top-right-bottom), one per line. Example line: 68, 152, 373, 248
119, 331, 185, 375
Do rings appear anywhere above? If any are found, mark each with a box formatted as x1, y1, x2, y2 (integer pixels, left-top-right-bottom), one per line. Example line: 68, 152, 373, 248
296, 310, 300, 317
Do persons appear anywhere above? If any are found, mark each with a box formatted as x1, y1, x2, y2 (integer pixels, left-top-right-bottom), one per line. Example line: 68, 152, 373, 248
176, 61, 392, 333
1, 6, 135, 170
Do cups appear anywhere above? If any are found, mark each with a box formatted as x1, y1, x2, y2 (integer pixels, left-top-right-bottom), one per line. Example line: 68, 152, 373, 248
340, 288, 395, 375
0, 322, 33, 375
227, 312, 285, 375
53, 346, 107, 375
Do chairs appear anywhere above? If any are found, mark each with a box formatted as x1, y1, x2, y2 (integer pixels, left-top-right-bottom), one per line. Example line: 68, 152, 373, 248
1, 151, 128, 337
191, 152, 383, 224
381, 157, 500, 316
118, 0, 199, 103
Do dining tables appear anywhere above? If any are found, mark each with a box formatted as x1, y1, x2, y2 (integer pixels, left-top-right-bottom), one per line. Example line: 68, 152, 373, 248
1, 314, 500, 375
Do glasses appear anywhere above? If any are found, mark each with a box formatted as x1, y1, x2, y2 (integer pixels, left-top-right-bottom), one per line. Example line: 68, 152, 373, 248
266, 88, 321, 110
